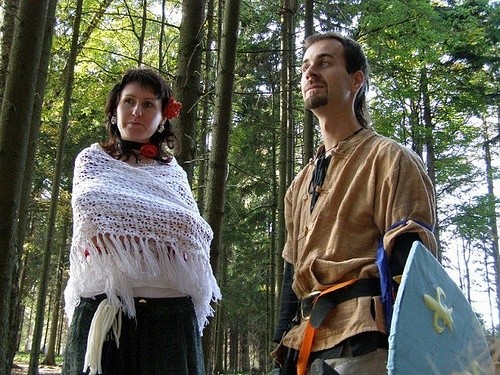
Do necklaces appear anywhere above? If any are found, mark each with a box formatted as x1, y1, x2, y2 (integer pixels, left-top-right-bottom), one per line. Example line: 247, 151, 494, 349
319, 125, 366, 155
119, 138, 158, 158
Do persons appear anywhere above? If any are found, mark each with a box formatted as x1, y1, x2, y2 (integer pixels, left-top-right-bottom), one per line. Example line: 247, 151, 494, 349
63, 69, 222, 374
271, 31, 437, 375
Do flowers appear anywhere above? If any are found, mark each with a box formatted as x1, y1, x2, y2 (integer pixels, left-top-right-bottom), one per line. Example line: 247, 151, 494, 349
141, 145, 157, 157
162, 96, 183, 119
